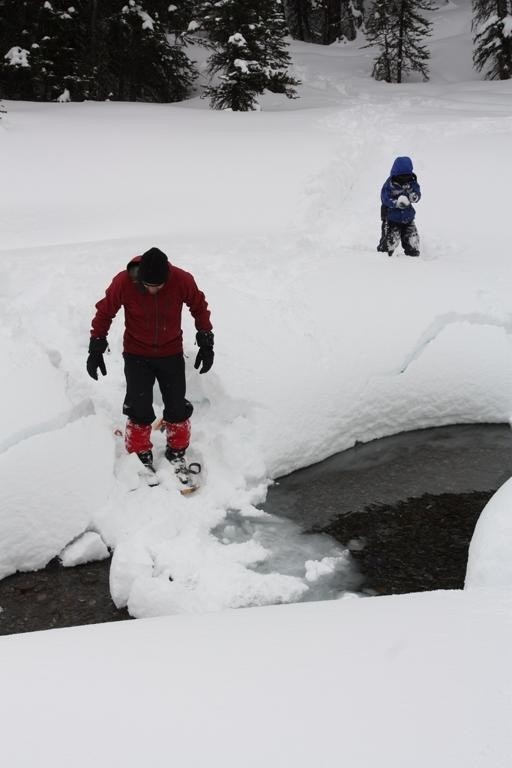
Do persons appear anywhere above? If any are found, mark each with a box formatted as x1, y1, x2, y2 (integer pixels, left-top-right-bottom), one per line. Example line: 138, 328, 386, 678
375, 157, 423, 258
86, 247, 216, 483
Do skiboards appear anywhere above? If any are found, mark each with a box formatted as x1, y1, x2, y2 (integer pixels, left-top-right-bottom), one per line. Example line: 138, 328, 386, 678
114, 419, 201, 496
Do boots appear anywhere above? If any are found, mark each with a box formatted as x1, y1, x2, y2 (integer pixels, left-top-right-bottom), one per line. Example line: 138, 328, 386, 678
166, 447, 190, 483
138, 450, 155, 476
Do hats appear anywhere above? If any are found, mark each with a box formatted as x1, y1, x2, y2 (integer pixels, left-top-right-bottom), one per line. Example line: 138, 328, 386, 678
139, 248, 168, 283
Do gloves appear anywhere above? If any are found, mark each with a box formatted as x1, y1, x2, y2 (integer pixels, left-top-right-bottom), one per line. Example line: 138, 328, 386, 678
194, 332, 214, 374
87, 339, 108, 381
